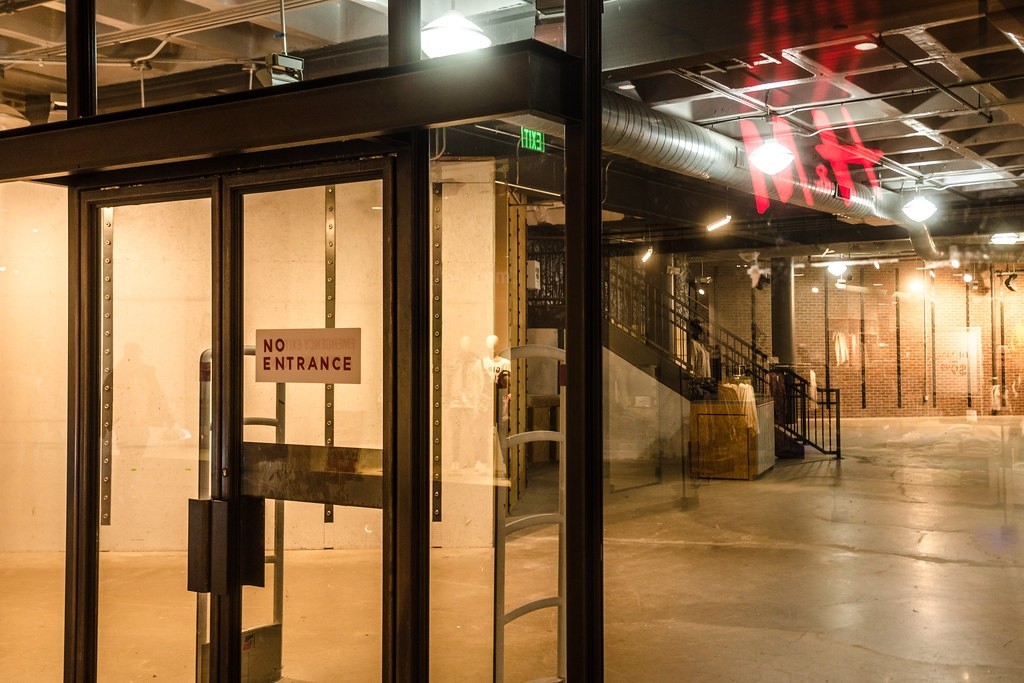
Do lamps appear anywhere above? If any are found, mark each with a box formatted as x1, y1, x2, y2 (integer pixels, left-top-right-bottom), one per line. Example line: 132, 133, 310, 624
421, 0, 492, 59
901, 186, 937, 223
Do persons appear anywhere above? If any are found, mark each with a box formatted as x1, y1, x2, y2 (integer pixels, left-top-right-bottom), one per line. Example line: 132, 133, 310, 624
443, 335, 511, 474
689, 329, 712, 381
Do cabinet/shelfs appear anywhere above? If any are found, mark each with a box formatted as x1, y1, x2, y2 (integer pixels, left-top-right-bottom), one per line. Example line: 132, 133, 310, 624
690, 396, 776, 481
